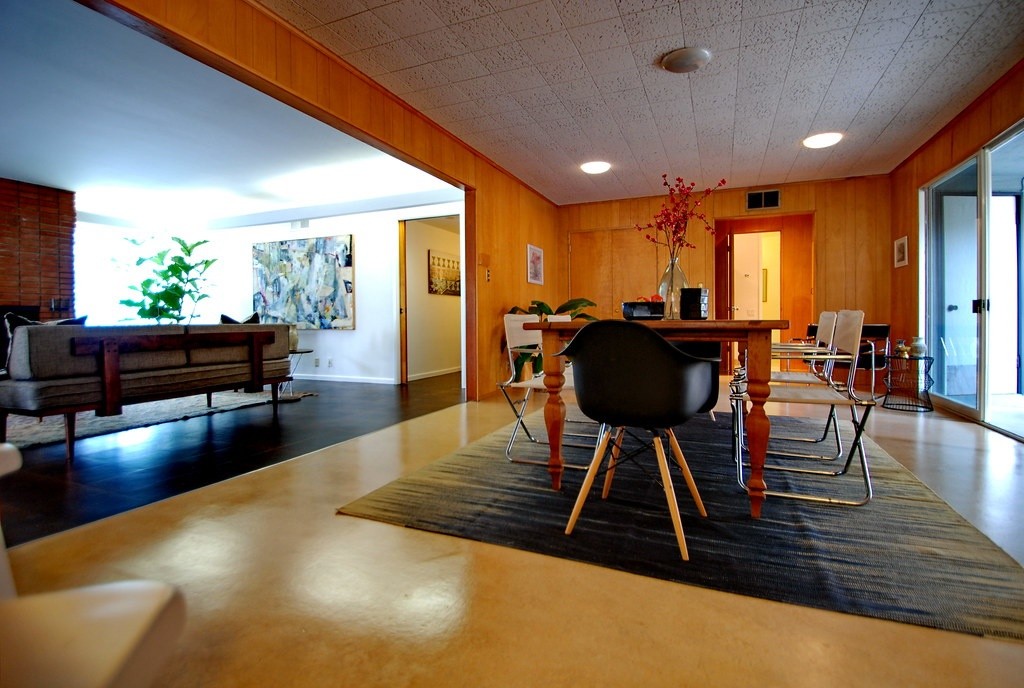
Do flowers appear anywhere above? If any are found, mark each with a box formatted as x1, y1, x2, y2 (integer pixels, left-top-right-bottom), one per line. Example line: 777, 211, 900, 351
633, 173, 727, 317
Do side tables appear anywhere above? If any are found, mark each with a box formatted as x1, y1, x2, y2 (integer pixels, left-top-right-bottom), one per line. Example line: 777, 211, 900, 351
881, 355, 935, 413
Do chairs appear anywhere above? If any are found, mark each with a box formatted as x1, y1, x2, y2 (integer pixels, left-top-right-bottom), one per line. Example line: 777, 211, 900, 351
0, 442, 189, 688
498, 309, 893, 509
552, 320, 722, 562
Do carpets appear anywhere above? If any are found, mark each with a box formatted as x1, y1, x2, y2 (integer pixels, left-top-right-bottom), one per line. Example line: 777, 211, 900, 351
2, 389, 318, 449
338, 400, 1024, 644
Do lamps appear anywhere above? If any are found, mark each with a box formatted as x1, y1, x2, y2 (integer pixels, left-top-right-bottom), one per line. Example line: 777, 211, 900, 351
662, 47, 711, 74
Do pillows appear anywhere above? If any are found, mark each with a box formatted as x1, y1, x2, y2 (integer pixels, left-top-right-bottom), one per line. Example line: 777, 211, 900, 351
220, 311, 261, 325
184, 323, 291, 367
8, 325, 187, 381
4, 312, 89, 375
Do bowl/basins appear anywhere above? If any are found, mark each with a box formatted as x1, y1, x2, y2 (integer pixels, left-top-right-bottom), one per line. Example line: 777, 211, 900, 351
622, 301, 665, 320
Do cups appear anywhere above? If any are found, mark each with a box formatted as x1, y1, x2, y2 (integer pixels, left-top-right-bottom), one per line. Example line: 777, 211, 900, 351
679, 287, 709, 320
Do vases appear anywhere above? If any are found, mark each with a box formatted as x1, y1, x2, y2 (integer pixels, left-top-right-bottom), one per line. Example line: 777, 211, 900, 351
659, 256, 689, 318
895, 336, 928, 359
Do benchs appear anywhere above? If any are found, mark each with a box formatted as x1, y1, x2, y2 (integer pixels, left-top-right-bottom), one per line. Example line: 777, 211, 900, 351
0, 324, 292, 462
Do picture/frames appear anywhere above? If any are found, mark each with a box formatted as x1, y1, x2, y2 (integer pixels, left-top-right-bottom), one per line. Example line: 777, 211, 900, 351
893, 235, 908, 269
527, 244, 544, 286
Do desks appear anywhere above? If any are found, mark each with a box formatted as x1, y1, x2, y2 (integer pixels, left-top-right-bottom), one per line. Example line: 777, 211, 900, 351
278, 349, 314, 398
523, 319, 791, 522
